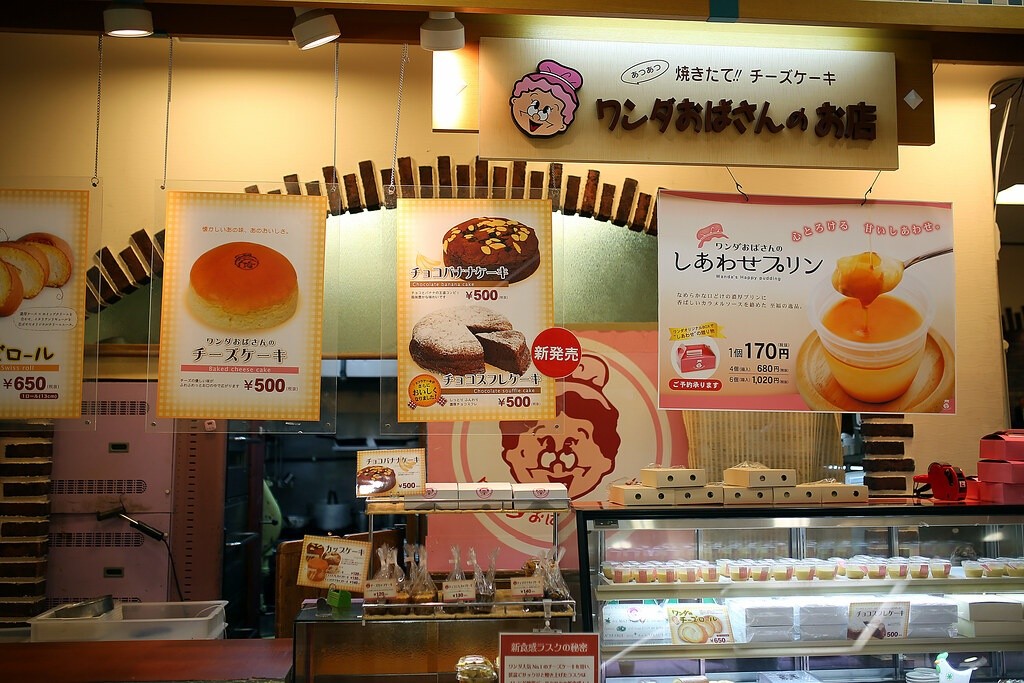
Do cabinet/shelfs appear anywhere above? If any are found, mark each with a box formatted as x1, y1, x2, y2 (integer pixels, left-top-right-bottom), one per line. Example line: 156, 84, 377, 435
572, 501, 1024, 683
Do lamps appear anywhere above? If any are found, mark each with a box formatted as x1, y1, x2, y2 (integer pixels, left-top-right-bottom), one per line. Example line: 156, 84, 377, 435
103, 9, 154, 38
420, 11, 465, 51
292, 6, 341, 52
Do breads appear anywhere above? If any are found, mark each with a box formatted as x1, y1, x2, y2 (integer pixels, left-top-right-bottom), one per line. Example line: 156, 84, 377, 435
677, 615, 723, 642
521, 558, 571, 614
0, 232, 74, 318
441, 217, 541, 285
847, 618, 887, 641
362, 577, 496, 614
406, 304, 532, 377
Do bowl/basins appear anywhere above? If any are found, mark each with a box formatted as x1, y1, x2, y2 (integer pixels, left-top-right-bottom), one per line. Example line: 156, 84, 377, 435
54, 594, 114, 619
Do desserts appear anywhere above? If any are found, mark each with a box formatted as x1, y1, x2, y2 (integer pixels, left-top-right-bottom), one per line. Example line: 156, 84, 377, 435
305, 542, 341, 582
185, 241, 301, 331
601, 554, 1024, 583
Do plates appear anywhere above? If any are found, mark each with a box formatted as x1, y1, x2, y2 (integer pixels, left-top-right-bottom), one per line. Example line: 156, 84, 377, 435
794, 328, 955, 413
905, 668, 939, 683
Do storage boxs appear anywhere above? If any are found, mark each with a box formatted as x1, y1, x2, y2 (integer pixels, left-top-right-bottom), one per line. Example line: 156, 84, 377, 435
725, 595, 1024, 642
511, 483, 568, 510
979, 429, 1024, 460
965, 475, 979, 500
979, 481, 1024, 504
977, 459, 1024, 484
608, 468, 868, 505
404, 483, 458, 510
457, 482, 513, 509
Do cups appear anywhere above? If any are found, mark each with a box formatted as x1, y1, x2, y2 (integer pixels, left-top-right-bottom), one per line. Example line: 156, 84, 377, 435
806, 271, 932, 403
